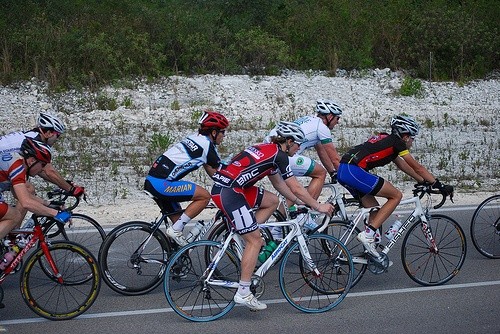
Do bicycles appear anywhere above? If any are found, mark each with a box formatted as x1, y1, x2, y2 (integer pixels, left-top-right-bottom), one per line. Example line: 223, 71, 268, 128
164, 194, 354, 321
300, 181, 467, 295
98, 170, 382, 296
470, 196, 500, 259
0, 181, 107, 321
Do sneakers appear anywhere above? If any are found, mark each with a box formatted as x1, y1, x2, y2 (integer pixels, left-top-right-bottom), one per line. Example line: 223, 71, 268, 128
296, 214, 318, 231
357, 231, 380, 257
234, 292, 267, 309
4, 234, 35, 248
166, 226, 194, 251
159, 259, 187, 279
366, 260, 393, 270
270, 227, 288, 240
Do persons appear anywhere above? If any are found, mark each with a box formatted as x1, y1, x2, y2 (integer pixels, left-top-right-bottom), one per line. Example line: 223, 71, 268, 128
338, 115, 454, 273
211, 121, 335, 311
0, 112, 85, 247
143, 110, 229, 279
263, 101, 342, 241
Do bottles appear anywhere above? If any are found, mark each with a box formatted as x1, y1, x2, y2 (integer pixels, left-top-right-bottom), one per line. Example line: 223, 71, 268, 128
0, 246, 20, 271
384, 219, 402, 240
289, 206, 298, 219
185, 220, 204, 243
20, 218, 35, 237
258, 239, 279, 264
375, 228, 381, 243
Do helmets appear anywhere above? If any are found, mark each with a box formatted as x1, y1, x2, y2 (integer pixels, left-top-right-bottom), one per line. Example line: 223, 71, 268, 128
275, 121, 308, 142
198, 112, 230, 131
390, 114, 419, 136
316, 100, 342, 115
18, 136, 54, 164
38, 112, 64, 134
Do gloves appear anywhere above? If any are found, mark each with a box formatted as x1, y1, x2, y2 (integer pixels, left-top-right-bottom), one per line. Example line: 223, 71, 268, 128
330, 170, 338, 180
418, 180, 428, 188
432, 179, 454, 196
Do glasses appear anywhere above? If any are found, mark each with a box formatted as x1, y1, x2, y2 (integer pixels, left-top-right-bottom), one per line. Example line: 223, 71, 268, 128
337, 115, 341, 120
294, 141, 303, 146
36, 159, 47, 167
410, 136, 415, 140
216, 130, 225, 134
56, 134, 60, 138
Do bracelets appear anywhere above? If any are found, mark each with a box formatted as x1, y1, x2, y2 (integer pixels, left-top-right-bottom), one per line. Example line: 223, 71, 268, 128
296, 199, 302, 205
293, 197, 297, 203
314, 202, 321, 210
